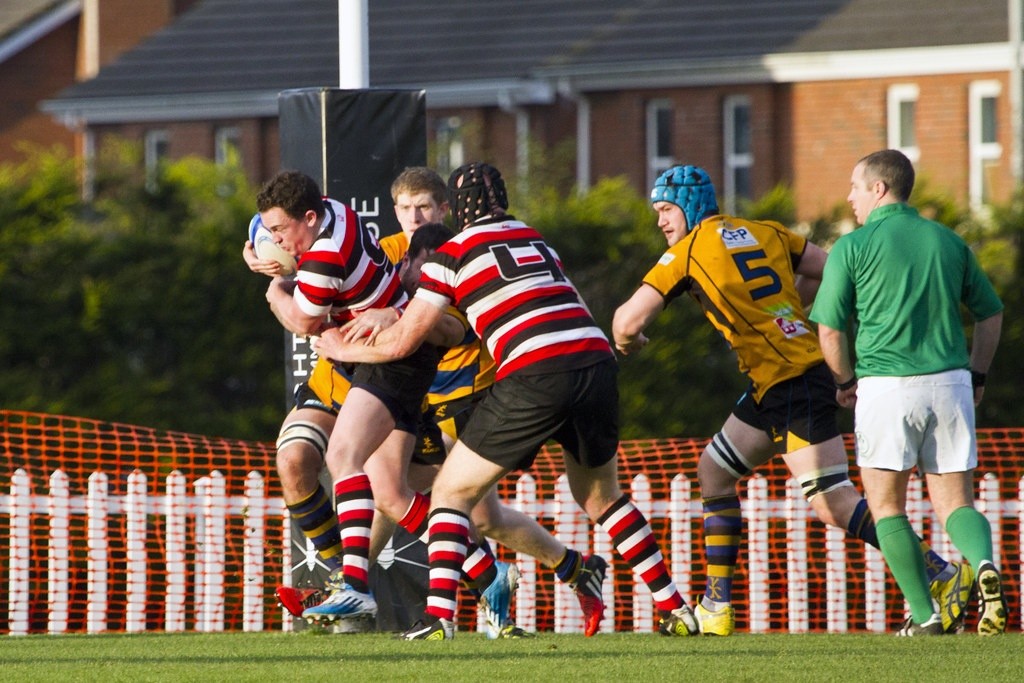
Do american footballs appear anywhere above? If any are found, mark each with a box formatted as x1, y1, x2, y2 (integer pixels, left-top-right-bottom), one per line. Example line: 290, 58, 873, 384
248, 211, 296, 281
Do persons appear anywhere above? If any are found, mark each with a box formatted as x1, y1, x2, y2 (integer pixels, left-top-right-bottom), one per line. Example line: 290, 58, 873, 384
807, 149, 1007, 635
312, 160, 699, 641
242, 167, 539, 643
612, 164, 977, 638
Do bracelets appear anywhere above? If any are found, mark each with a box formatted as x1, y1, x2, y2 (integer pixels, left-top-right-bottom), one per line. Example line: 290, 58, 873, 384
834, 375, 857, 391
970, 371, 987, 387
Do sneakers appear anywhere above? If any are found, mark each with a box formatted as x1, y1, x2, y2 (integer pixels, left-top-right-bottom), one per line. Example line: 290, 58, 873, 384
574, 555, 610, 636
976, 559, 1009, 636
477, 560, 522, 640
302, 583, 378, 619
656, 603, 699, 637
391, 618, 454, 640
894, 613, 945, 636
325, 620, 368, 632
501, 615, 525, 640
276, 587, 333, 619
928, 560, 974, 633
693, 603, 735, 636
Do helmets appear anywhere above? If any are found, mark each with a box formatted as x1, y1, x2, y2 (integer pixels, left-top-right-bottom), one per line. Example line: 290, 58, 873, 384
446, 162, 509, 234
651, 165, 719, 231
